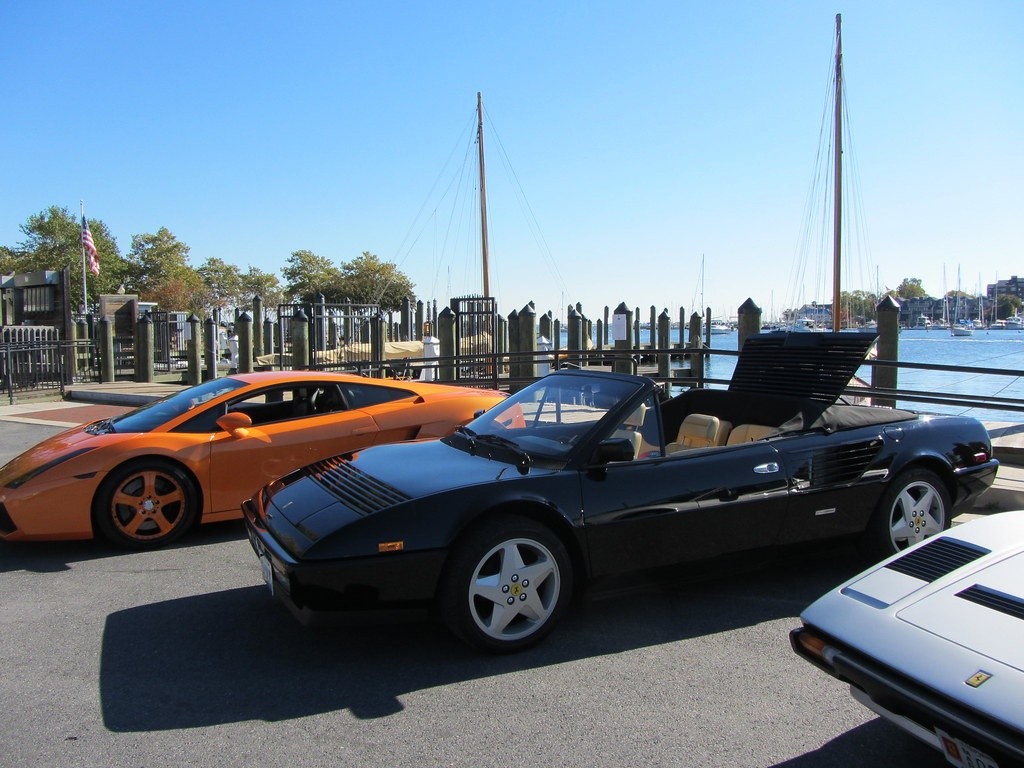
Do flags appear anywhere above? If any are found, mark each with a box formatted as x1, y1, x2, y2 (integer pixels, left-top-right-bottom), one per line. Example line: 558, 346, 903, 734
80, 213, 101, 277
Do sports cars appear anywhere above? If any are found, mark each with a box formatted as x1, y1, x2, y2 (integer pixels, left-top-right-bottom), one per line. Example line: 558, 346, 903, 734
0, 371, 525, 551
238, 327, 1001, 656
790, 510, 1024, 768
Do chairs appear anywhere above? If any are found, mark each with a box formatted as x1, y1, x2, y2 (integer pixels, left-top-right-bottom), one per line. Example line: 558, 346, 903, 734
607, 402, 644, 464
663, 414, 777, 456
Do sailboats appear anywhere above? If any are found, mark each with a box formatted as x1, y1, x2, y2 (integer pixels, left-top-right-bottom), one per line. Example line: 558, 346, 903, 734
700, 12, 1024, 358
255, 91, 510, 374
